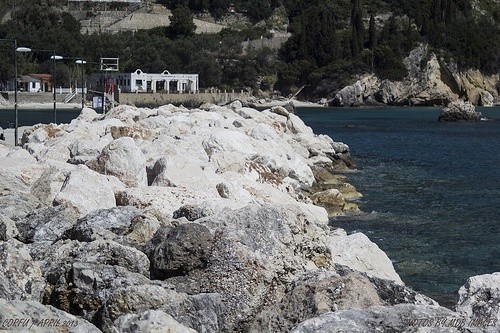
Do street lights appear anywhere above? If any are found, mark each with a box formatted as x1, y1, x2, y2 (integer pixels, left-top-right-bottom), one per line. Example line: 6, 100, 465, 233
50, 55, 84, 111
16, 46, 56, 124
76, 59, 106, 114
